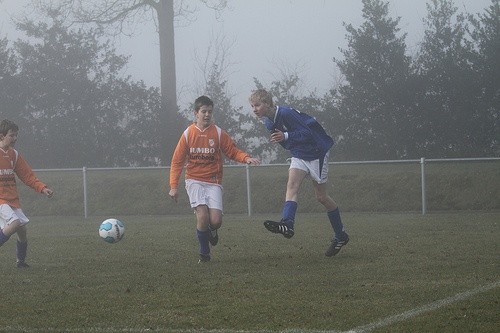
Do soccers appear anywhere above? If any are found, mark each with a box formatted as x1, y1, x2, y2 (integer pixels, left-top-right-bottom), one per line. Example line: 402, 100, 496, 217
99, 218, 126, 244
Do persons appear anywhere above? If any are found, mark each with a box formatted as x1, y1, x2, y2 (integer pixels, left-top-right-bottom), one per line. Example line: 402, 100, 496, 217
168, 96, 260, 262
0, 120, 55, 269
249, 90, 349, 257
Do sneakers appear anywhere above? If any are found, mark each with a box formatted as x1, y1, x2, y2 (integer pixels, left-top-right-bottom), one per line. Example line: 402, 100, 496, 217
264, 219, 295, 239
325, 234, 350, 256
197, 254, 211, 264
209, 229, 218, 246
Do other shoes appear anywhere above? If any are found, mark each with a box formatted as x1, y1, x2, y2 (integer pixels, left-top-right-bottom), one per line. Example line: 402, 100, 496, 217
17, 261, 29, 269
